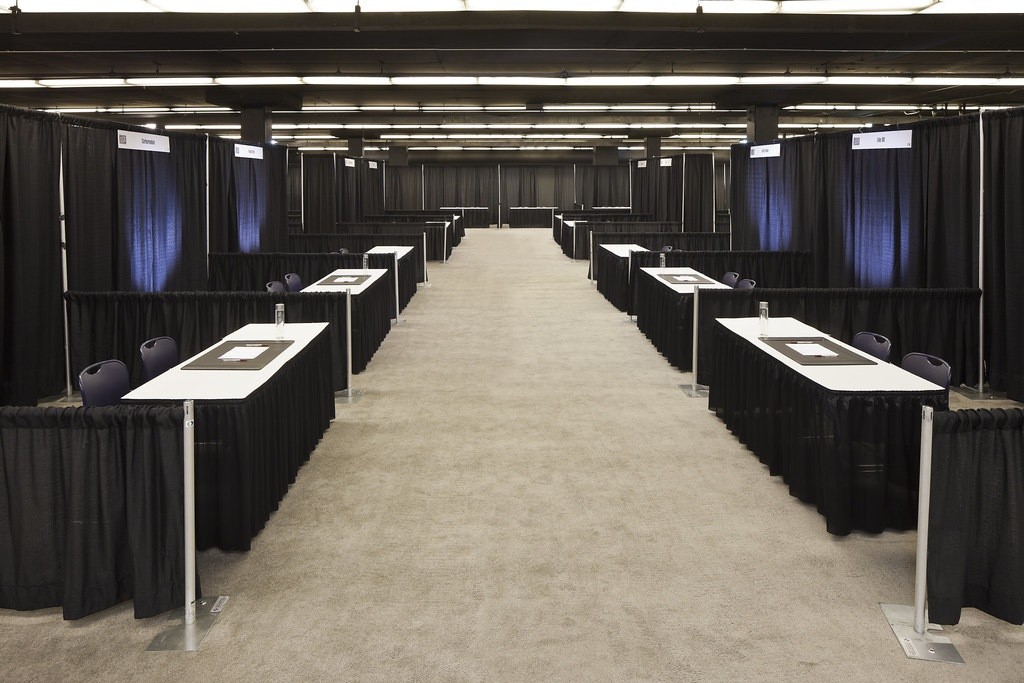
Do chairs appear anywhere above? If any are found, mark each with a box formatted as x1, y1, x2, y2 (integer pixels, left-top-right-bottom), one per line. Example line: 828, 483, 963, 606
139, 336, 179, 383
737, 279, 756, 289
340, 248, 350, 254
285, 273, 302, 292
266, 280, 285, 293
901, 353, 951, 388
723, 272, 739, 288
662, 246, 673, 251
852, 332, 891, 362
80, 360, 131, 408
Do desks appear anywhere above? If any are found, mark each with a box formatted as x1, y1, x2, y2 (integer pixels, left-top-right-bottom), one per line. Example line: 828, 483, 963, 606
562, 221, 588, 258
121, 322, 335, 529
708, 317, 949, 538
365, 246, 417, 314
509, 207, 558, 228
440, 207, 489, 228
426, 222, 452, 260
554, 214, 561, 245
593, 206, 631, 211
298, 268, 391, 374
454, 215, 465, 237
596, 244, 653, 312
636, 267, 733, 372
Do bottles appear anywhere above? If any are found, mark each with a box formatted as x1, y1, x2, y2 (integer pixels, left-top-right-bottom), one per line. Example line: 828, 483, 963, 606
362, 253, 368, 276
659, 252, 665, 276
274, 303, 285, 339
758, 301, 768, 336
